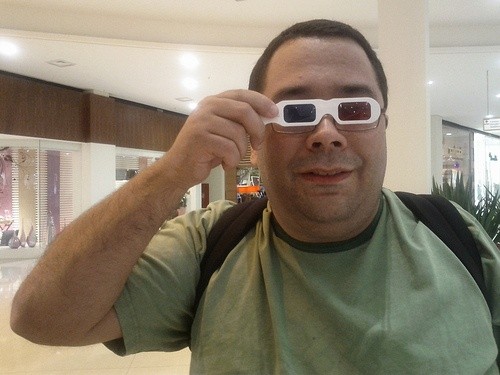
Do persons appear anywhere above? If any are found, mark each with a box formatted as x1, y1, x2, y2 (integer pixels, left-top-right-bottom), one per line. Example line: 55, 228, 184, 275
8, 17, 500, 375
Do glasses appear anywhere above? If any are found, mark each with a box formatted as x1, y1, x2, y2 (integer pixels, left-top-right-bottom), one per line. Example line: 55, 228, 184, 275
260, 96, 386, 134
